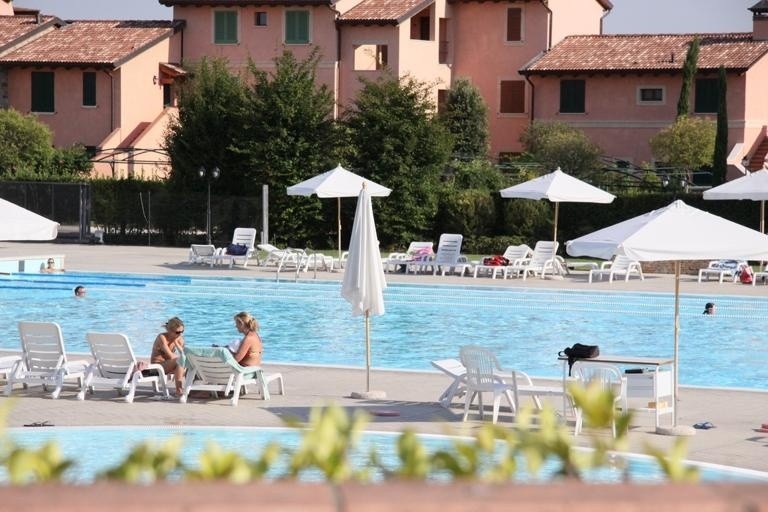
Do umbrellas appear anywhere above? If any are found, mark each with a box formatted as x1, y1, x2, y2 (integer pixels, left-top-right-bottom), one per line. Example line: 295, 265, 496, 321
0, 196, 62, 242
702, 167, 768, 235
499, 168, 617, 245
563, 197, 768, 439
283, 164, 393, 261
340, 182, 388, 399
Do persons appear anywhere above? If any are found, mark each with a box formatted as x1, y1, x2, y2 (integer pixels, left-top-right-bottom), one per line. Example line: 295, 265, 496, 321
74, 285, 89, 298
149, 317, 186, 399
188, 310, 263, 398
698, 301, 717, 316
40, 257, 65, 274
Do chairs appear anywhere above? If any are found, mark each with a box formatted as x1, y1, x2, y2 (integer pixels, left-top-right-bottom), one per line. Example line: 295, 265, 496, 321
698, 257, 768, 289
187, 226, 349, 273
458, 343, 517, 426
563, 363, 630, 440
427, 348, 583, 430
381, 233, 644, 284
79, 332, 176, 403
0, 313, 91, 399
178, 344, 287, 407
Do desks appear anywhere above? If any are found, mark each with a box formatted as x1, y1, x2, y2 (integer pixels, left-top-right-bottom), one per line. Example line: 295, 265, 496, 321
558, 354, 679, 433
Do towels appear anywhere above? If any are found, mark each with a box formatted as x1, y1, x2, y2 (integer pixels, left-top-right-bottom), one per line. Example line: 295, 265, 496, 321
179, 345, 263, 379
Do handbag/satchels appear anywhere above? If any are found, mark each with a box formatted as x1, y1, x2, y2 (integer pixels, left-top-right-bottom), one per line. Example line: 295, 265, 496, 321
559, 343, 600, 360
